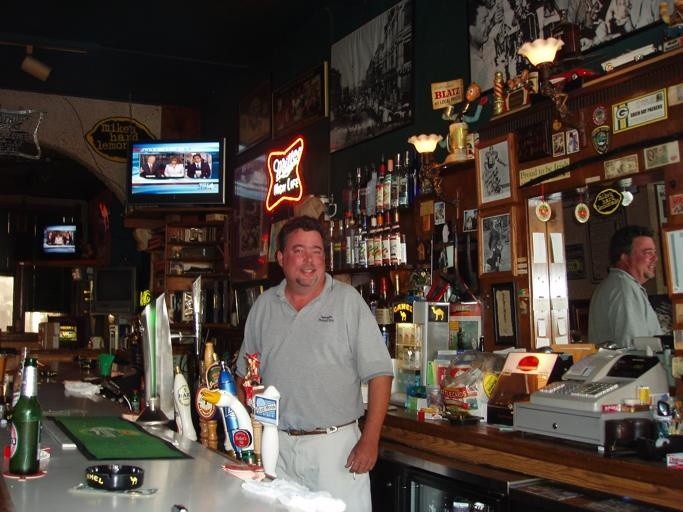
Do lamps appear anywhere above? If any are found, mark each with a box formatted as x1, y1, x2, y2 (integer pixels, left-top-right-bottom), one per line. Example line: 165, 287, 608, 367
617, 177, 634, 207
407, 131, 442, 192
516, 35, 574, 119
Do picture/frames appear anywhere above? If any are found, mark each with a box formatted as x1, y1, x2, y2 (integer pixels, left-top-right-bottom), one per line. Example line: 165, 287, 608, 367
465, 1, 676, 98
271, 60, 328, 137
474, 207, 518, 279
563, 242, 588, 281
490, 281, 520, 349
232, 79, 274, 157
473, 131, 518, 209
327, 1, 416, 157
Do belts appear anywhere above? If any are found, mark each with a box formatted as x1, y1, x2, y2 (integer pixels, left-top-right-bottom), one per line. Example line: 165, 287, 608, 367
283, 420, 355, 436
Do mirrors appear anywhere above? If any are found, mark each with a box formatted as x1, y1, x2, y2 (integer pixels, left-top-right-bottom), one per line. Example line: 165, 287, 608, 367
512, 131, 682, 366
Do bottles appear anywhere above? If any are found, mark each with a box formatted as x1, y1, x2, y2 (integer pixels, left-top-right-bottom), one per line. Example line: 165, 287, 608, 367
10, 355, 40, 477
332, 206, 408, 272
343, 148, 425, 217
130, 394, 140, 413
359, 273, 407, 349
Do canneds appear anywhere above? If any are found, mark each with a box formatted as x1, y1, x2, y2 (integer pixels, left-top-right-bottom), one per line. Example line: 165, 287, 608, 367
449, 122, 469, 153
470, 132, 481, 156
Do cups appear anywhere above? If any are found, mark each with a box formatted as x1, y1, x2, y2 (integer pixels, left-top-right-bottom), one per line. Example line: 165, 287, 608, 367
97, 352, 115, 377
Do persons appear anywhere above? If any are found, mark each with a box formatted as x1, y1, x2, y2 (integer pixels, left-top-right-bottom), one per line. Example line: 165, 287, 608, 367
47, 231, 72, 245
488, 219, 503, 271
456, 327, 464, 352
587, 224, 664, 349
482, 147, 507, 193
234, 214, 395, 512
467, 2, 505, 94
139, 153, 210, 179
337, 93, 411, 126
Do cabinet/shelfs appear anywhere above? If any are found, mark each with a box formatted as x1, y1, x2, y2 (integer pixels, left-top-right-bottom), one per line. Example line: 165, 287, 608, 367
121, 207, 227, 328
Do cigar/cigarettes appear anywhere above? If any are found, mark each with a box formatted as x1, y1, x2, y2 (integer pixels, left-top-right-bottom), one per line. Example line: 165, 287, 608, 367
351, 461, 356, 480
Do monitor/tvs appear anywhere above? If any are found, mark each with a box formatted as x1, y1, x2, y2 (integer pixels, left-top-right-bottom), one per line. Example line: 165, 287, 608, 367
94, 266, 136, 312
43, 225, 77, 254
127, 138, 226, 204
